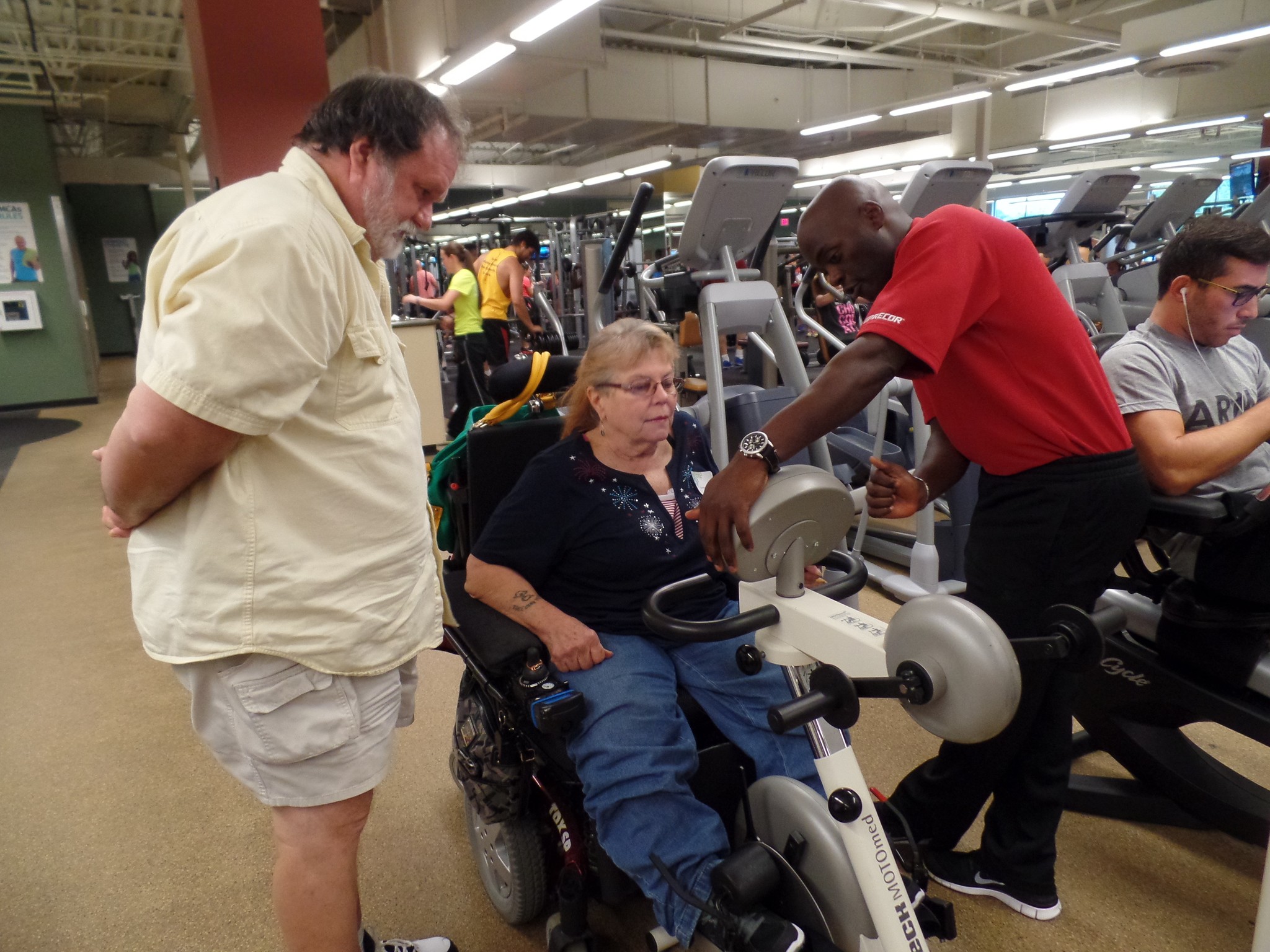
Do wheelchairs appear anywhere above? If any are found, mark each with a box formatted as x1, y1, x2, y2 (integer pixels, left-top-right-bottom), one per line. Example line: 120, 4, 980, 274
423, 352, 742, 929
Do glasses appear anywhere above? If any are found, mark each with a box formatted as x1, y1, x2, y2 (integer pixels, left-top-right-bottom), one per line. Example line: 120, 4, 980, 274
1189, 274, 1270, 306
592, 377, 685, 398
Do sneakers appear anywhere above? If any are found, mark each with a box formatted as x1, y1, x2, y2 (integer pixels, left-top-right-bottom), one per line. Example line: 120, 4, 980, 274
900, 874, 925, 910
356, 927, 459, 951
922, 848, 1062, 920
696, 889, 804, 952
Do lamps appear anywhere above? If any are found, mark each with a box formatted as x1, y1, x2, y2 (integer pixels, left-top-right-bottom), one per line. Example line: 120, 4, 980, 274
415, 0, 1269, 214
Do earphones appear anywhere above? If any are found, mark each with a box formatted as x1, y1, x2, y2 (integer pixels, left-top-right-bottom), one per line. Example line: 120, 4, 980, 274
1180, 287, 1187, 305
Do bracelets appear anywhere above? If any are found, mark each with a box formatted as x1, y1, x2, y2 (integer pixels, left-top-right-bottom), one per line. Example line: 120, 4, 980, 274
910, 474, 929, 510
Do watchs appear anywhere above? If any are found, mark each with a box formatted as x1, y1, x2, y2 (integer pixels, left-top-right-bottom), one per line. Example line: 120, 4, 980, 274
737, 432, 783, 476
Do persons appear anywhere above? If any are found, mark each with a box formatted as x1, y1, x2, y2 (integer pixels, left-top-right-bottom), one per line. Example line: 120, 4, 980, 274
87, 69, 471, 952
1095, 211, 1270, 702
409, 259, 440, 320
9, 235, 43, 283
473, 226, 545, 372
467, 316, 852, 952
121, 251, 142, 281
695, 179, 1151, 922
401, 238, 489, 444
522, 233, 875, 386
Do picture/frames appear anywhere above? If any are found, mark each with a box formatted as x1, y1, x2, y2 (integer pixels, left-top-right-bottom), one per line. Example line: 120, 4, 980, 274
0, 290, 43, 332
